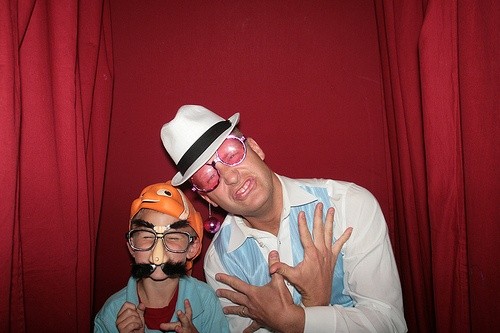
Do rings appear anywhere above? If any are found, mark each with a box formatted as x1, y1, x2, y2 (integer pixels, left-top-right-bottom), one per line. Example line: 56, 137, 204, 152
241, 306, 248, 316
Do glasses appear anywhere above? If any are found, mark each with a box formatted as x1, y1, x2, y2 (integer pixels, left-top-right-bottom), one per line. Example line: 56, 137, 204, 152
191, 135, 246, 193
125, 228, 198, 253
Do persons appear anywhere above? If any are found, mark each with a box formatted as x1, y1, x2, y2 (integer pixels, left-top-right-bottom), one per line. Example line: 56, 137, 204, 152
159, 104, 409, 333
94, 179, 232, 333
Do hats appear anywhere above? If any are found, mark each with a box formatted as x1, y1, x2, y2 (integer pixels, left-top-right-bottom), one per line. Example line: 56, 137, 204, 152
160, 105, 240, 187
127, 180, 203, 276
